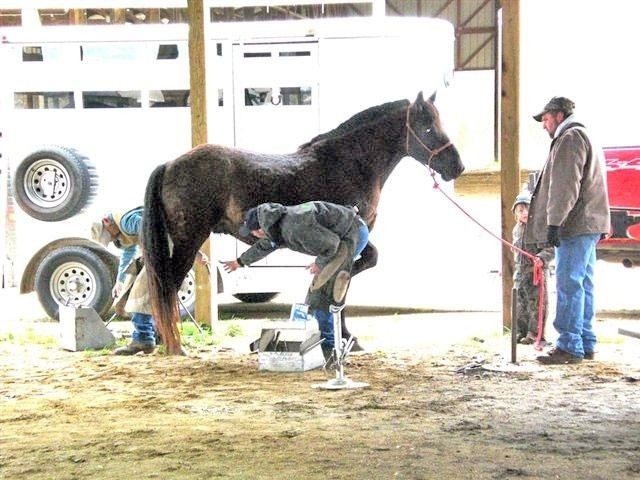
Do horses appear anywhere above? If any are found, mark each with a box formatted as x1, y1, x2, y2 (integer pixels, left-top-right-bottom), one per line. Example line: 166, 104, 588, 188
139, 90, 466, 356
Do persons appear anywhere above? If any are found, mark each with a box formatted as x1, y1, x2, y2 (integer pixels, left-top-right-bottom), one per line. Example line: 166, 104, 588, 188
91, 204, 210, 357
219, 203, 368, 368
524, 96, 611, 364
510, 191, 555, 344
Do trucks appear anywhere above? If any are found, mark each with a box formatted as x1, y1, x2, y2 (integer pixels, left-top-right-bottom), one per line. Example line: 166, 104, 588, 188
0, 8, 455, 322
593, 144, 640, 269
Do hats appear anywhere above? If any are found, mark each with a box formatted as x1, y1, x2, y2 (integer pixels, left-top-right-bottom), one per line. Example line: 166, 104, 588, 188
239, 207, 261, 238
91, 222, 112, 248
533, 96, 575, 122
513, 194, 531, 210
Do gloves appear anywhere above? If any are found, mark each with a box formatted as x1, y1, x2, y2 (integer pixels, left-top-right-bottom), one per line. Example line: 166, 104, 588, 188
546, 225, 560, 248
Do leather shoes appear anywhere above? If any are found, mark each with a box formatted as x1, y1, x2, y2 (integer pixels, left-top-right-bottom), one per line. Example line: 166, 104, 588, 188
547, 346, 595, 360
115, 340, 156, 356
535, 347, 582, 364
